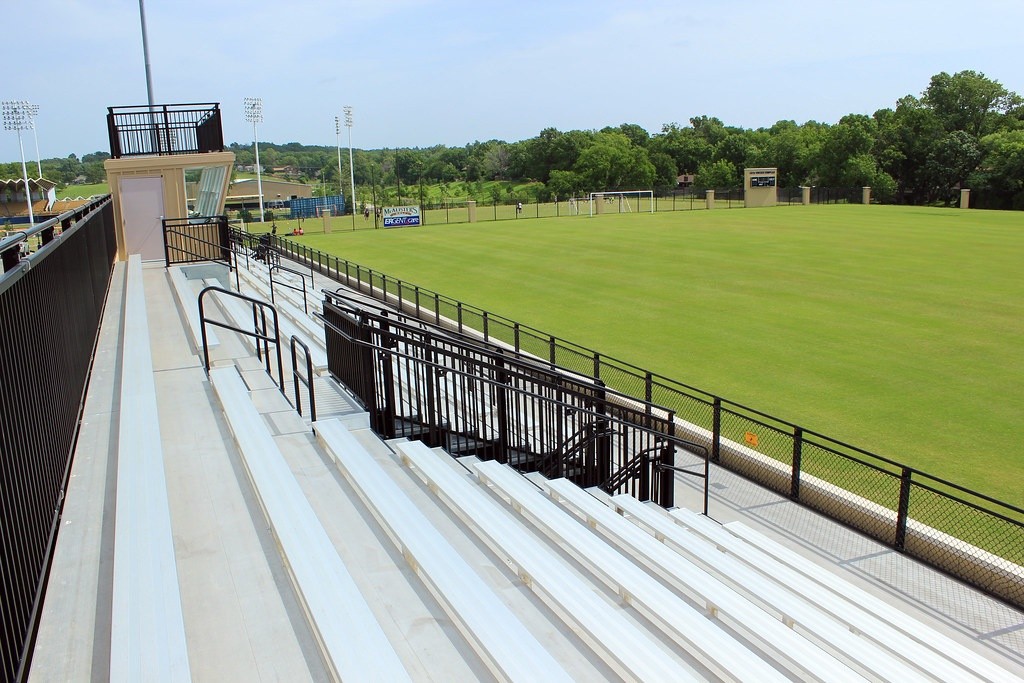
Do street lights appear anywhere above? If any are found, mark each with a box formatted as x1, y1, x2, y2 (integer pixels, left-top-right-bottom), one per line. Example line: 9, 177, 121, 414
1, 100, 35, 228
30, 103, 43, 177
244, 96, 265, 223
341, 104, 357, 230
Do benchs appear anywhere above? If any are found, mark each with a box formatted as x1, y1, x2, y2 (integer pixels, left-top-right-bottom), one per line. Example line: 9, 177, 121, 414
166, 248, 1024, 683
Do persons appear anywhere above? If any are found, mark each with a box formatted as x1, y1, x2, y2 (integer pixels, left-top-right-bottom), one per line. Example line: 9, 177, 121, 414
333, 209, 383, 223
272, 225, 278, 236
292, 228, 305, 236
517, 196, 558, 214
